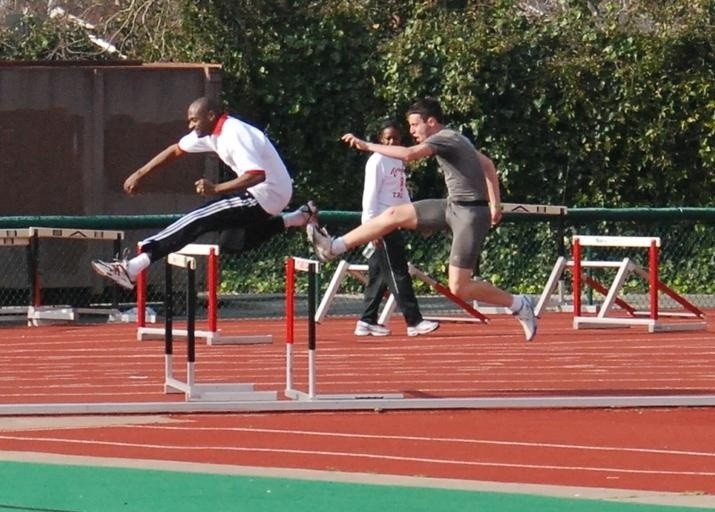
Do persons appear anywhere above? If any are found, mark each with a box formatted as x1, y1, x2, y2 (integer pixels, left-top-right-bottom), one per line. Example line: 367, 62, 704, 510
352, 119, 441, 338
304, 99, 537, 343
90, 92, 322, 292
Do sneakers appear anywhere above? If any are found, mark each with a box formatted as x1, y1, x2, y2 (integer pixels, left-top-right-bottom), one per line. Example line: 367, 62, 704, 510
407, 320, 439, 337
354, 320, 390, 336
92, 259, 136, 291
302, 200, 335, 262
512, 294, 538, 342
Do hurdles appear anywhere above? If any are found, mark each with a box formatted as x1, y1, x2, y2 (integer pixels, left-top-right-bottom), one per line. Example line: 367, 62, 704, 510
176, 242, 274, 345
138, 242, 185, 341
0, 228, 157, 328
533, 236, 707, 333
163, 252, 278, 402
285, 255, 406, 402
314, 260, 491, 330
